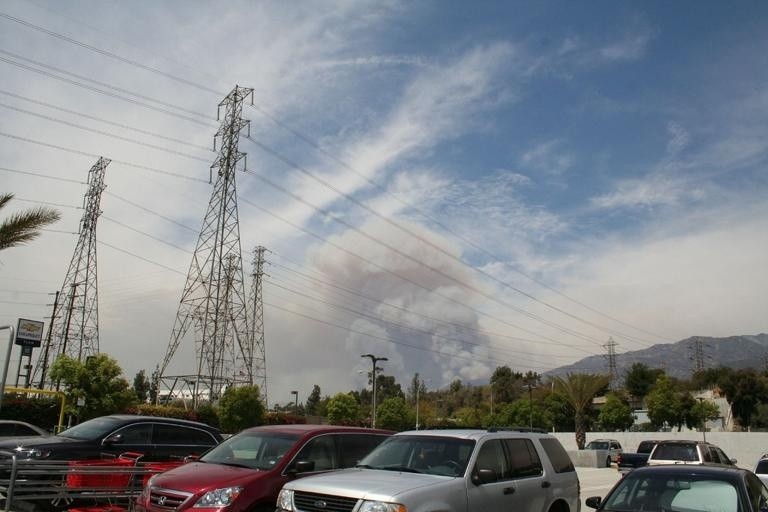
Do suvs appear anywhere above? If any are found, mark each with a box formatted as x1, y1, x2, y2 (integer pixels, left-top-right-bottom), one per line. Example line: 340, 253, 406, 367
0, 414, 235, 512
136, 425, 419, 511
274, 427, 582, 512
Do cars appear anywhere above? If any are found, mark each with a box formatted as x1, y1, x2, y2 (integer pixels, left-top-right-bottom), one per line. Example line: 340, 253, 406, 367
753, 453, 768, 486
584, 464, 768, 512
645, 439, 738, 470
584, 439, 623, 468
0, 420, 53, 439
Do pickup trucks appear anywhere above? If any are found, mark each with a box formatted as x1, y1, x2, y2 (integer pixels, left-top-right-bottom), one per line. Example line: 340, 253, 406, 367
616, 438, 660, 478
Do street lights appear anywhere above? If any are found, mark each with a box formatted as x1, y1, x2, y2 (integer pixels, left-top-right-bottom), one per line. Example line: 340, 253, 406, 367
416, 379, 432, 430
358, 354, 388, 428
291, 391, 298, 415
